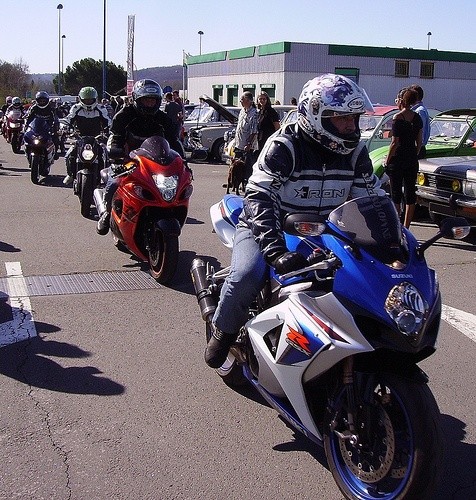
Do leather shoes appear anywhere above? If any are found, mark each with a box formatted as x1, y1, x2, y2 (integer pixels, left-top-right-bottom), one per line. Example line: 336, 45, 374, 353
95, 210, 111, 236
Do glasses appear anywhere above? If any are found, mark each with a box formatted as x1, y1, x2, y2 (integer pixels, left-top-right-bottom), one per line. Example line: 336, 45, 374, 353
395, 98, 404, 103
259, 98, 267, 100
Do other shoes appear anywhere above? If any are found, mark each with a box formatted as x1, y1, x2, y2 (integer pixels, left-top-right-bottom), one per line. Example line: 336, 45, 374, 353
62, 175, 74, 185
204, 329, 238, 369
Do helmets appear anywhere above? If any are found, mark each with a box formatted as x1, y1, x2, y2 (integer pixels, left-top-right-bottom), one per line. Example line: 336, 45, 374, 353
131, 78, 163, 117
78, 86, 98, 110
56, 98, 63, 105
36, 91, 50, 108
12, 97, 22, 108
6, 96, 12, 105
297, 73, 367, 155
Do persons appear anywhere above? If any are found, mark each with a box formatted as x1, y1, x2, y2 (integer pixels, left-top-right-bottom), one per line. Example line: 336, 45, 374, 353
274, 100, 284, 120
22, 91, 59, 160
94, 79, 189, 236
0, 90, 184, 175
203, 72, 389, 369
384, 84, 431, 228
221, 91, 280, 188
59, 87, 112, 185
290, 96, 297, 105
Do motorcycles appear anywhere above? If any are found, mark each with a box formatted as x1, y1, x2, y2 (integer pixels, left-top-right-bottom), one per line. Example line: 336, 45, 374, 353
94, 136, 194, 286
58, 118, 111, 217
0, 108, 76, 184
189, 191, 471, 500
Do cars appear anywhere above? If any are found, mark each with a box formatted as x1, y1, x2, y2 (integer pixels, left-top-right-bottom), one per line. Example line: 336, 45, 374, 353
158, 92, 298, 164
402, 156, 476, 241
358, 106, 442, 153
368, 108, 476, 198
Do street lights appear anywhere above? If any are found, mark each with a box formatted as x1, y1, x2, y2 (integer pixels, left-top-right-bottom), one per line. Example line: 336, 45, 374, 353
56, 3, 63, 96
427, 30, 432, 50
197, 30, 204, 55
61, 35, 67, 72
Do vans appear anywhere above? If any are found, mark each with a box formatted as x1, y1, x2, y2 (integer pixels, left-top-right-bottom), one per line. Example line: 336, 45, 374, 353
48, 94, 80, 107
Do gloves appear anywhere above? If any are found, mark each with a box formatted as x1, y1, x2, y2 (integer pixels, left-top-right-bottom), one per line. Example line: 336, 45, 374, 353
111, 162, 128, 177
275, 252, 309, 277
